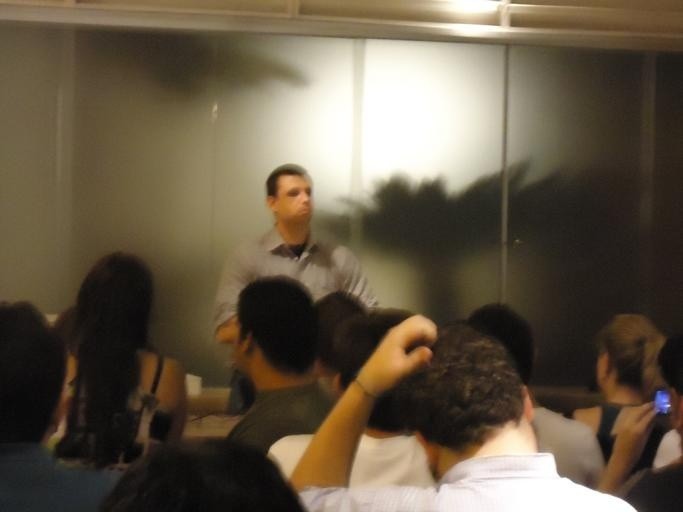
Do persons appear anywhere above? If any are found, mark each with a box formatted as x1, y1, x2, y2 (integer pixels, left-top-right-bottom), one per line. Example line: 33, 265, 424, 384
212, 160, 379, 414
1, 252, 683, 512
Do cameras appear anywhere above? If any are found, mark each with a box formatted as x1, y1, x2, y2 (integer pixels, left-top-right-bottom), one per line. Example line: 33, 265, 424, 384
652, 387, 671, 417
